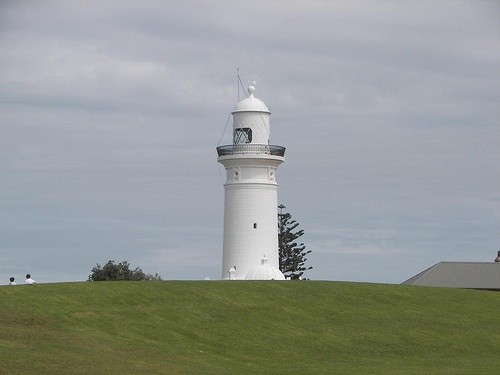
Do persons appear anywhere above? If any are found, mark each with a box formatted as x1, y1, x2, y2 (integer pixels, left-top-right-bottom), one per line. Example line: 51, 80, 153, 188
25, 274, 35, 284
9, 277, 16, 286
